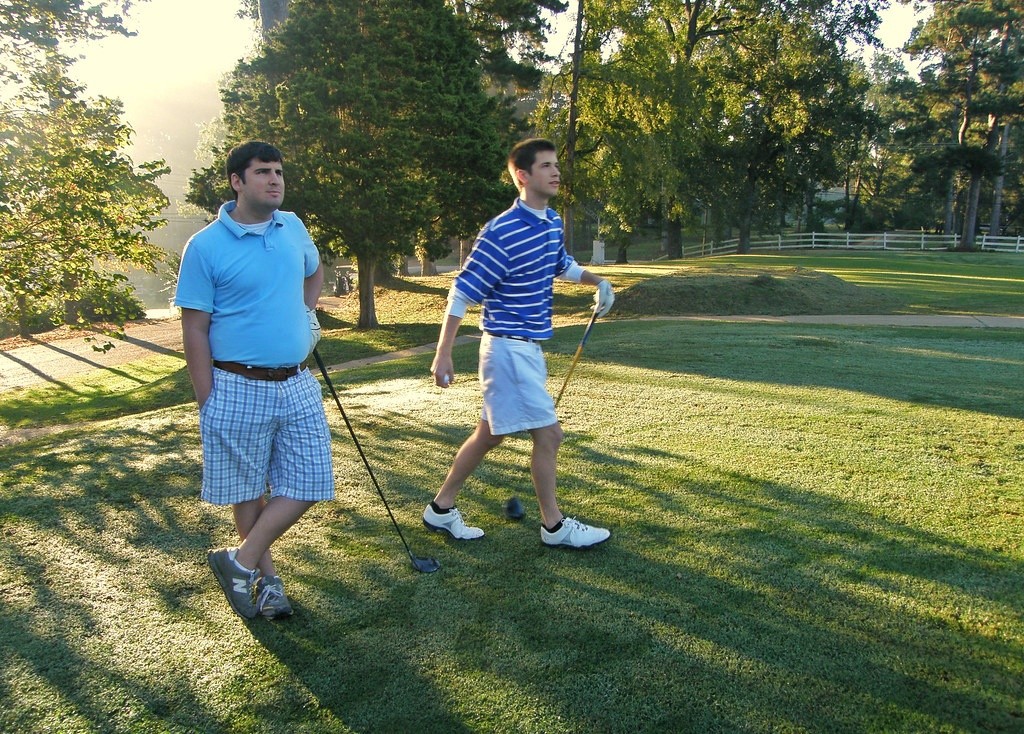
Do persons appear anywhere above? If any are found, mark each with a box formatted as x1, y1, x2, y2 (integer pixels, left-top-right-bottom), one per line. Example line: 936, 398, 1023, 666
173, 139, 336, 629
421, 137, 616, 550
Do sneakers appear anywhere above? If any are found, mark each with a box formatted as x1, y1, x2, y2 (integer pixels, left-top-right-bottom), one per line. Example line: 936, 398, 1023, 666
255, 575, 294, 621
541, 515, 611, 548
423, 504, 485, 540
207, 548, 261, 620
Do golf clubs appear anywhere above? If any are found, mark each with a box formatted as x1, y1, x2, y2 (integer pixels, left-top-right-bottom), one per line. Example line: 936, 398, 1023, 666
506, 312, 600, 519
312, 348, 440, 574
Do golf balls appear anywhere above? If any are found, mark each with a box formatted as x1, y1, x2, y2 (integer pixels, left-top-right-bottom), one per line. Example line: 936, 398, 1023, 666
444, 374, 450, 384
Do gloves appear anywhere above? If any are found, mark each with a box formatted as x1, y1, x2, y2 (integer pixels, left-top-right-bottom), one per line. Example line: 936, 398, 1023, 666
305, 304, 322, 355
590, 279, 615, 319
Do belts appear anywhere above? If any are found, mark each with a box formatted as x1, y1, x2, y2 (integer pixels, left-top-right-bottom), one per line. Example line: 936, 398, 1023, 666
488, 330, 537, 342
212, 359, 307, 381
